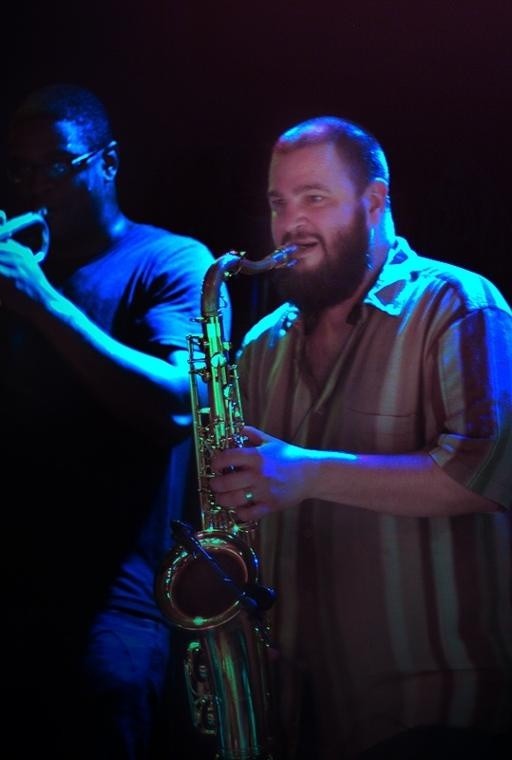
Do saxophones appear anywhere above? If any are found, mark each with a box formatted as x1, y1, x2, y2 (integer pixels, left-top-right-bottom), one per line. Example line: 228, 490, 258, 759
152, 245, 300, 760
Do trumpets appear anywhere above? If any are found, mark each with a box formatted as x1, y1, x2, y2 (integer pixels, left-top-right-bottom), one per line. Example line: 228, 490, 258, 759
1, 209, 51, 264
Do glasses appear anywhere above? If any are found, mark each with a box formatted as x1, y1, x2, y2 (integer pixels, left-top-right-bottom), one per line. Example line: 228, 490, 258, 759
48, 140, 116, 179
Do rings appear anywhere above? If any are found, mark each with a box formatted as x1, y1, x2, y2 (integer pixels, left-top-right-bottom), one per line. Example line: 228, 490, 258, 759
244, 490, 255, 500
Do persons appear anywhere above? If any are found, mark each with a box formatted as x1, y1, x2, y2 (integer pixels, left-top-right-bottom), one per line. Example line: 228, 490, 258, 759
1, 82, 233, 760
206, 115, 510, 759
